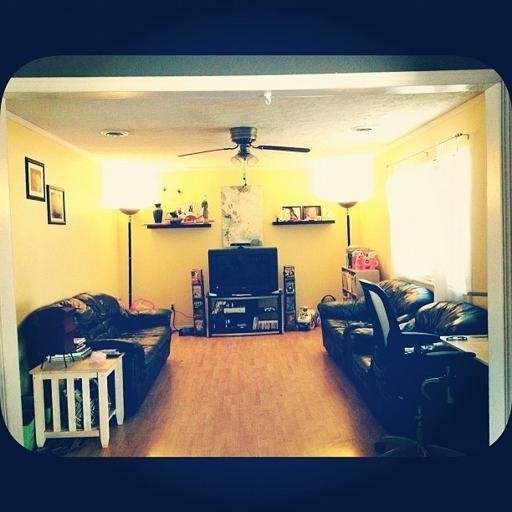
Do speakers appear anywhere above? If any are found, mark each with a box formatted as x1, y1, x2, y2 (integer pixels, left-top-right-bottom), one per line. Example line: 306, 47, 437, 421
191, 269, 206, 336
284, 265, 296, 331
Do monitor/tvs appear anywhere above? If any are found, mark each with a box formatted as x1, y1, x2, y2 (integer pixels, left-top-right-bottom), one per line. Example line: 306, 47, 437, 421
208, 247, 278, 295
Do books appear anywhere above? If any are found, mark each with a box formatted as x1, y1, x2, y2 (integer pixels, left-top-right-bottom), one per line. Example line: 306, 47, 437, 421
45, 336, 92, 361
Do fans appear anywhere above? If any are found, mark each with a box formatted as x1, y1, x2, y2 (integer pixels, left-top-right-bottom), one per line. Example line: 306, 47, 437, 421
176, 126, 313, 168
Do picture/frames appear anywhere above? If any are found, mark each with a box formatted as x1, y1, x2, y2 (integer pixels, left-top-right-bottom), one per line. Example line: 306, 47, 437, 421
24, 156, 68, 226
282, 204, 322, 222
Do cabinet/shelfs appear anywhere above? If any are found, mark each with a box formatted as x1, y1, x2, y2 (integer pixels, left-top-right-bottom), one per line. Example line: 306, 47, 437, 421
204, 294, 283, 337
341, 266, 380, 300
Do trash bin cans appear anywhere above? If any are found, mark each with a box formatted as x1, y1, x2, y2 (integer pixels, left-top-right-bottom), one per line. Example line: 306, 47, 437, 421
22, 394, 36, 452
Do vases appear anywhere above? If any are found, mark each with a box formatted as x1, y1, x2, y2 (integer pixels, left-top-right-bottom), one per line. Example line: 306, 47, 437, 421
153, 202, 164, 223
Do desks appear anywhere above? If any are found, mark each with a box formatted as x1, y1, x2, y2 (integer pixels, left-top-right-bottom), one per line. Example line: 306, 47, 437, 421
28, 351, 127, 448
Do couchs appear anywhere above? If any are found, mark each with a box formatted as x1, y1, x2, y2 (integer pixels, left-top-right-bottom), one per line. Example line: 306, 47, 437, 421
316, 279, 487, 436
15, 292, 173, 424
